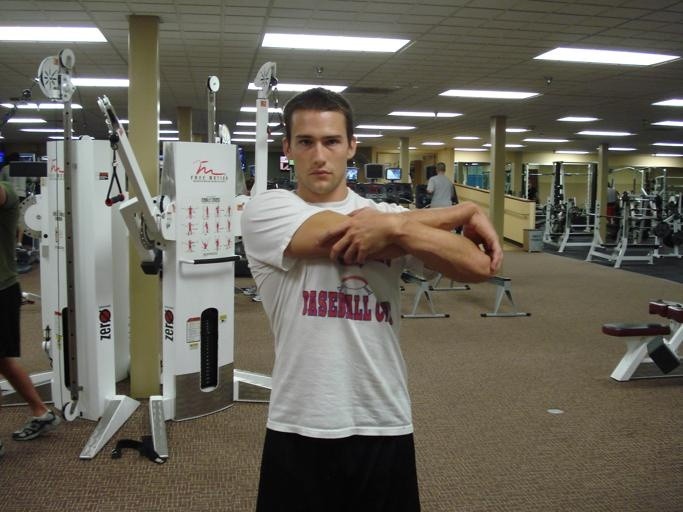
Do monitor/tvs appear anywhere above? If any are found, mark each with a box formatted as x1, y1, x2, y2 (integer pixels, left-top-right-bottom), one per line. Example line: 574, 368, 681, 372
18, 153, 35, 162
345, 167, 358, 181
364, 164, 385, 179
279, 155, 291, 171
386, 168, 401, 180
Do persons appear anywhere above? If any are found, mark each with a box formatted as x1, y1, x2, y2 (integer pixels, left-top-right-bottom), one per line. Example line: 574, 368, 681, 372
238, 88, 505, 510
0, 151, 31, 250
607, 182, 617, 225
425, 161, 454, 208
0, 173, 64, 441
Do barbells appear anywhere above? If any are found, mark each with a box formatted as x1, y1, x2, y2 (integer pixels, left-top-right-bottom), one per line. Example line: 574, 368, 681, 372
620, 191, 666, 232
543, 203, 581, 220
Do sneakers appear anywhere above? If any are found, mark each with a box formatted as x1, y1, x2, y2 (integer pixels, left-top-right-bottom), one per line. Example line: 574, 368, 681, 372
13, 410, 61, 441
